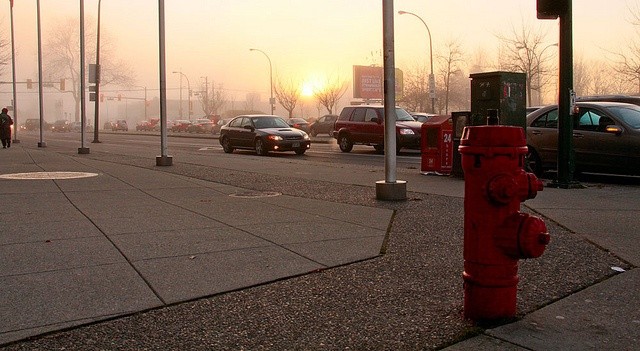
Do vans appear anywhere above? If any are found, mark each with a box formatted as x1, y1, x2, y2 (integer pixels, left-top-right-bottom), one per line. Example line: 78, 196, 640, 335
25, 119, 47, 130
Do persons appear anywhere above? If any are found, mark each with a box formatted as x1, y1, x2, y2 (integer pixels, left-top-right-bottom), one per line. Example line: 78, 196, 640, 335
1, 107, 13, 150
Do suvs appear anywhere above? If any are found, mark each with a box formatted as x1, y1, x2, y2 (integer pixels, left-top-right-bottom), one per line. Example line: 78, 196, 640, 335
333, 105, 424, 151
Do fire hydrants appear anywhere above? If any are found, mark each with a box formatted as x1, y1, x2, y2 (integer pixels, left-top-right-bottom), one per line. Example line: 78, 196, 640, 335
458, 109, 550, 318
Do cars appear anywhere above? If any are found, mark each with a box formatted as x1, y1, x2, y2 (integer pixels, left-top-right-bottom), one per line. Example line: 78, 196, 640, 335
211, 119, 230, 134
171, 120, 191, 132
52, 119, 72, 132
524, 101, 640, 178
308, 114, 338, 136
285, 118, 310, 130
136, 120, 151, 131
111, 119, 128, 131
219, 114, 310, 155
188, 119, 213, 133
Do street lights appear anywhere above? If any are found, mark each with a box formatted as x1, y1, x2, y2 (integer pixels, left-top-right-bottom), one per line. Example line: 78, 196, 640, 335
250, 48, 273, 114
398, 11, 434, 113
517, 43, 558, 105
172, 72, 190, 120
